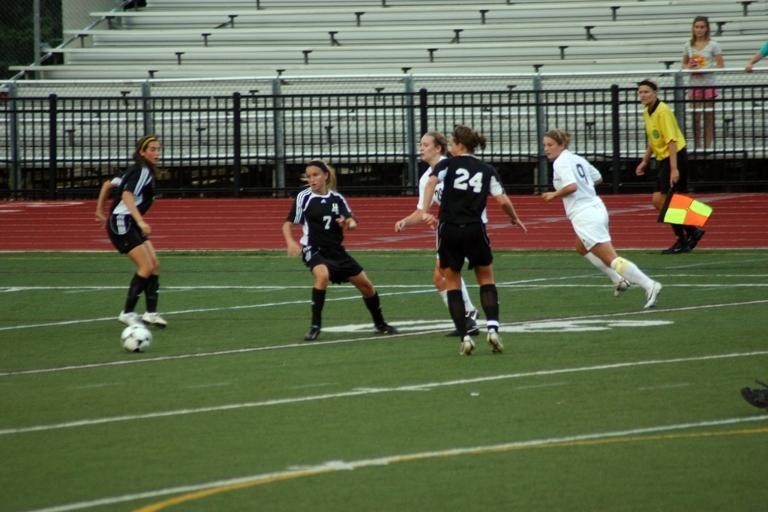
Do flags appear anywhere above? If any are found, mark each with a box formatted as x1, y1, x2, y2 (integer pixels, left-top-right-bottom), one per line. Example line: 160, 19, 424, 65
663, 189, 714, 228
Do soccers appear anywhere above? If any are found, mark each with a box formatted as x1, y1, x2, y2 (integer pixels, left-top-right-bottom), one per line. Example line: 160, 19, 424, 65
120, 326, 151, 352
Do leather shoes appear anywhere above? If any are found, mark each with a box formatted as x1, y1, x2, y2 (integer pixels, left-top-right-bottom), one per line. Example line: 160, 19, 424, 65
740, 382, 768, 412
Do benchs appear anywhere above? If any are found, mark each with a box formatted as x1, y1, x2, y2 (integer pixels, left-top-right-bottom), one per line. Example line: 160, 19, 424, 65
0, 1, 767, 149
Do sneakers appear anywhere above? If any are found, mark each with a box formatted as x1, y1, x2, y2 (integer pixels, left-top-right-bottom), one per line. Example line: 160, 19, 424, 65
642, 279, 663, 310
373, 323, 401, 335
458, 334, 476, 356
612, 279, 630, 298
661, 239, 692, 255
141, 310, 169, 330
445, 317, 480, 336
687, 226, 707, 249
303, 324, 321, 341
115, 310, 146, 331
485, 331, 504, 353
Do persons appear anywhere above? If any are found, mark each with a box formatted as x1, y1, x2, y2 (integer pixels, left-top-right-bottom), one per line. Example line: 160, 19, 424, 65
541, 128, 663, 309
421, 126, 530, 354
741, 378, 767, 410
681, 16, 730, 152
280, 160, 397, 341
93, 134, 167, 332
394, 131, 486, 335
634, 80, 707, 255
746, 39, 768, 73
